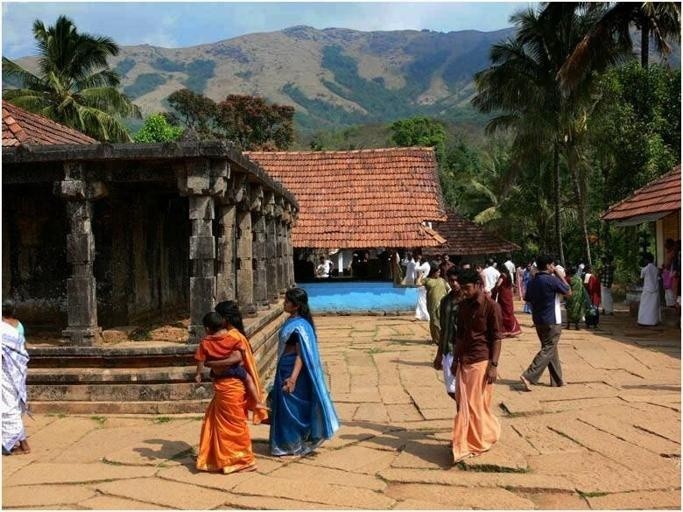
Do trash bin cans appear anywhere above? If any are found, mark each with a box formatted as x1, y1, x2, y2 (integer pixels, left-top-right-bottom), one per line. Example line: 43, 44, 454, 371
489, 360, 499, 369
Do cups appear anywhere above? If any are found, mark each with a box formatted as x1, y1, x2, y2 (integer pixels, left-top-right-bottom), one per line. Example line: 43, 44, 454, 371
449, 278, 457, 285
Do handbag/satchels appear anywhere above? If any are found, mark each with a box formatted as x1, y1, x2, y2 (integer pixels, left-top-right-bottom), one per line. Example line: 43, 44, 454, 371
287, 378, 296, 387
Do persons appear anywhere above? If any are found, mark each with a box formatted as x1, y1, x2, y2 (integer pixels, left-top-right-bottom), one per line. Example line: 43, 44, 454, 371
196, 312, 273, 413
661, 236, 681, 314
449, 268, 502, 465
192, 301, 268, 473
3, 297, 30, 456
637, 252, 661, 327
351, 248, 616, 342
316, 255, 334, 279
435, 265, 477, 449
267, 288, 341, 456
520, 254, 571, 392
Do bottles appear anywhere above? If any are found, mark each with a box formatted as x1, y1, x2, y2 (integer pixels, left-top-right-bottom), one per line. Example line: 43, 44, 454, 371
457, 269, 480, 285
